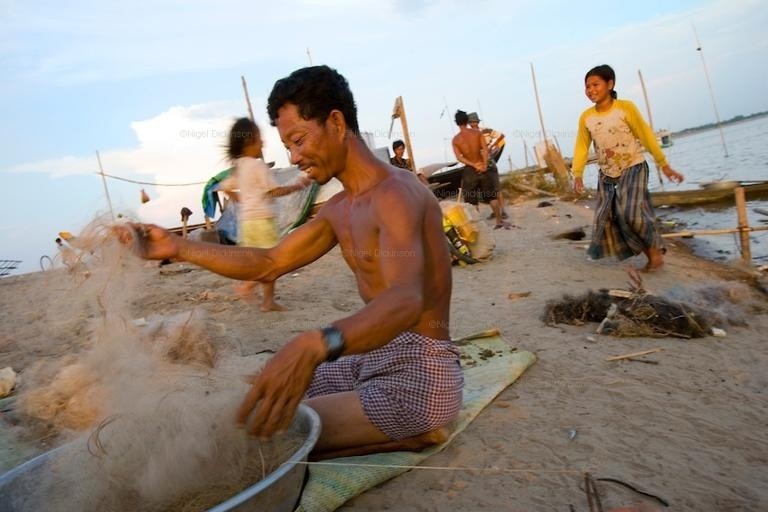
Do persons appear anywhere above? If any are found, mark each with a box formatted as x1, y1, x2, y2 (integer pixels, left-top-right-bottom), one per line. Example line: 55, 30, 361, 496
55, 238, 71, 271
466, 112, 509, 220
570, 62, 684, 274
113, 64, 465, 463
389, 139, 414, 172
218, 116, 312, 312
452, 109, 505, 231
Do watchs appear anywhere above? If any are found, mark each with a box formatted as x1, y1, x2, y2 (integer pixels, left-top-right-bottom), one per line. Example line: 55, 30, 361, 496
321, 323, 344, 362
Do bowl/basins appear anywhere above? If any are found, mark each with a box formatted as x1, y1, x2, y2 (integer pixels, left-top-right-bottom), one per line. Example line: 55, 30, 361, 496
0, 396, 324, 511
701, 180, 744, 191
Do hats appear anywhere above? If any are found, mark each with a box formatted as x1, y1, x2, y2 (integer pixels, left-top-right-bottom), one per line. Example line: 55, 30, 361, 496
181, 208, 192, 221
468, 112, 482, 122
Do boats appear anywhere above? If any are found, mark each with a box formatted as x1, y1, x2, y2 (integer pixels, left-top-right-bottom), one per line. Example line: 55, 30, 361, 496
59, 74, 505, 260
